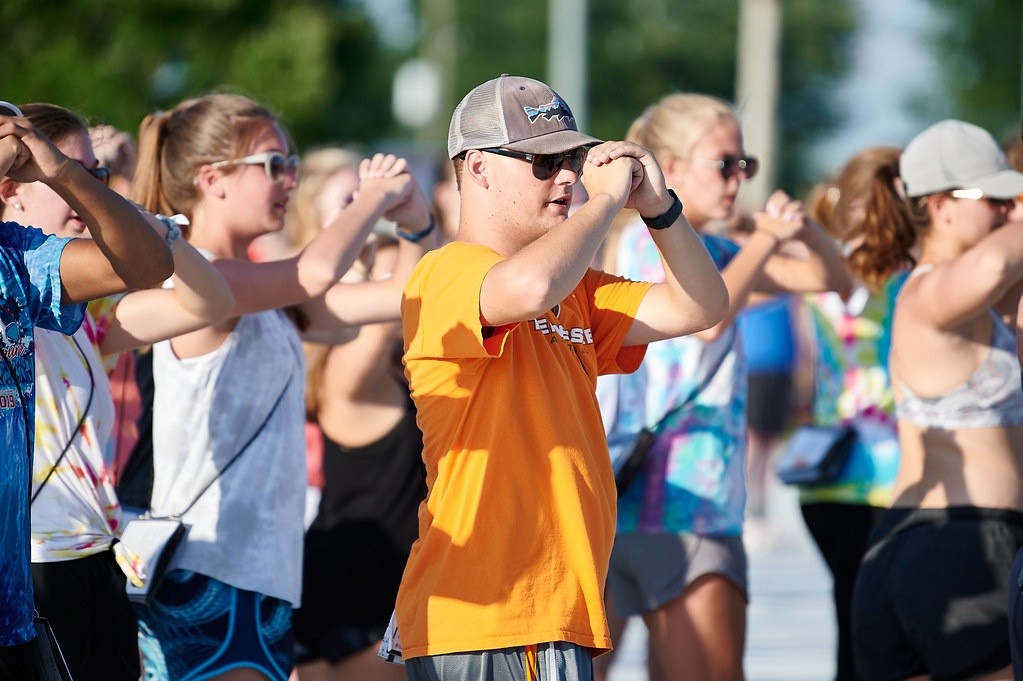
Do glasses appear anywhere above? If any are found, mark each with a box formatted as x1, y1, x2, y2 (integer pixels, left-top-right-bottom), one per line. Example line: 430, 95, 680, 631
675, 154, 761, 180
193, 153, 300, 186
85, 169, 108, 187
459, 145, 588, 181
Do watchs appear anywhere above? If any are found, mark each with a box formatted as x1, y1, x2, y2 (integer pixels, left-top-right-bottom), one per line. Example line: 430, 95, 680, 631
640, 189, 683, 230
156, 215, 181, 248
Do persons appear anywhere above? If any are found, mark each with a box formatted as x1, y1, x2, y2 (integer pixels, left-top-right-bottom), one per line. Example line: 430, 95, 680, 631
0, 101, 175, 681
247, 147, 430, 681
395, 74, 730, 681
832, 118, 1023, 680
81, 124, 154, 537
595, 95, 851, 681
798, 149, 902, 681
0, 104, 236, 680
724, 214, 794, 521
130, 94, 435, 680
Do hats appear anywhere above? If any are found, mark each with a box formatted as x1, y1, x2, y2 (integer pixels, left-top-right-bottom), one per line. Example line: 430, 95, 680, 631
898, 119, 1023, 198
448, 73, 606, 160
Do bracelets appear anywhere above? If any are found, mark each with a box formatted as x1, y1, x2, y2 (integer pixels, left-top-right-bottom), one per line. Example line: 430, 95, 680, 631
396, 212, 435, 243
